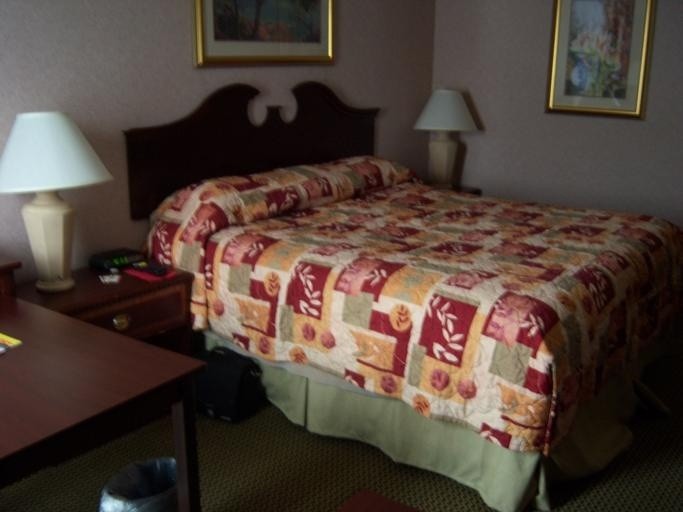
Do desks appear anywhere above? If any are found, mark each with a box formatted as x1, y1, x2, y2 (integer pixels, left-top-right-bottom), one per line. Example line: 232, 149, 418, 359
0, 291, 211, 510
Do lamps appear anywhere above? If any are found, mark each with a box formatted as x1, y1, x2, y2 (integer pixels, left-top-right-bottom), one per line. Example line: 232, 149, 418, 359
0, 111, 114, 294
410, 89, 477, 190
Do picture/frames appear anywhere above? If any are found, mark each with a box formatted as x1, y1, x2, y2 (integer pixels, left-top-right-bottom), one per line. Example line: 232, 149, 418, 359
192, 0, 337, 69
544, 0, 657, 121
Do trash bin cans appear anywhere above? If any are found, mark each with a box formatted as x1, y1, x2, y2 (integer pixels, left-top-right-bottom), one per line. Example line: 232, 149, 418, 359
104, 457, 178, 512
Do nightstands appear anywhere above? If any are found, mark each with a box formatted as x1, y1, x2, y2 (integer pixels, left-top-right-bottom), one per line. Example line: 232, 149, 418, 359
13, 248, 193, 358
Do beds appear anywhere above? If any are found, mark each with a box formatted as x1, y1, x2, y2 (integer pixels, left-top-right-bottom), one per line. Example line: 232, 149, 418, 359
120, 80, 683, 511
